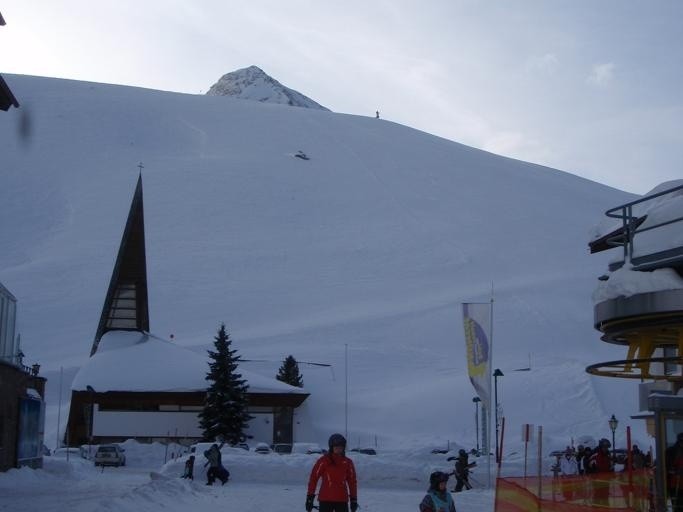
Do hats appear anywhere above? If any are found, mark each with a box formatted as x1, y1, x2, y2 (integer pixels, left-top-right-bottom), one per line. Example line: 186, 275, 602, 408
430, 470, 449, 492
563, 438, 611, 453
328, 432, 348, 458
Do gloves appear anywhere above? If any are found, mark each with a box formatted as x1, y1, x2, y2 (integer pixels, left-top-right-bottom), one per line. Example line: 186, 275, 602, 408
305, 493, 315, 512
350, 496, 358, 512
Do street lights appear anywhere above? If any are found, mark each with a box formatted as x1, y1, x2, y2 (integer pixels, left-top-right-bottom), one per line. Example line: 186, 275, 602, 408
491, 365, 504, 466
605, 412, 622, 476
468, 396, 482, 458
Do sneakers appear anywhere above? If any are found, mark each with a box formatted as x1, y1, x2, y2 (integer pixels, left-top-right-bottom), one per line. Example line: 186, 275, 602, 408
451, 486, 472, 492
205, 477, 229, 487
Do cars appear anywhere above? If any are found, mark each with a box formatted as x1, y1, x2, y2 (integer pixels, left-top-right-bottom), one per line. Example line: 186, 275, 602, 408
547, 445, 639, 467
430, 447, 492, 463
42, 443, 53, 458
305, 445, 378, 455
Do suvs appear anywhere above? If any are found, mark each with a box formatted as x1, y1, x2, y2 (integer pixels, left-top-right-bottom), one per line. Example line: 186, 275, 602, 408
94, 442, 129, 468
236, 442, 250, 454
254, 443, 271, 454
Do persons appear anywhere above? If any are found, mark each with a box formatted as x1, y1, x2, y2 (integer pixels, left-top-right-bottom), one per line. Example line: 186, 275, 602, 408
179, 454, 195, 481
205, 447, 226, 486
305, 433, 357, 511
449, 448, 473, 492
559, 436, 653, 475
209, 442, 229, 478
664, 432, 682, 512
418, 471, 456, 511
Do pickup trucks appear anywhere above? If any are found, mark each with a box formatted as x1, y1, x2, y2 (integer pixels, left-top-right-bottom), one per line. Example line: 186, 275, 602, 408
268, 444, 294, 456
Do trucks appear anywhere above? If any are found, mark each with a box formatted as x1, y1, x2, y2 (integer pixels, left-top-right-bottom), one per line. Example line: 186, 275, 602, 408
179, 442, 229, 459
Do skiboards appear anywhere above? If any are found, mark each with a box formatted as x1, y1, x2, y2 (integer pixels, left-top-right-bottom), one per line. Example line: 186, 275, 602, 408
453, 469, 472, 490
204, 437, 225, 467
448, 461, 477, 476
